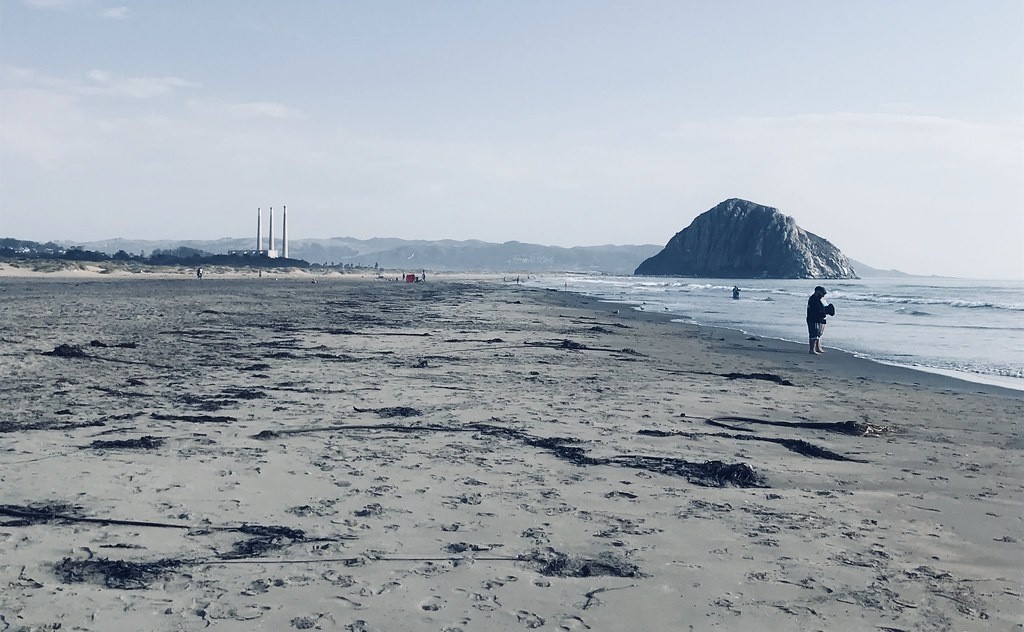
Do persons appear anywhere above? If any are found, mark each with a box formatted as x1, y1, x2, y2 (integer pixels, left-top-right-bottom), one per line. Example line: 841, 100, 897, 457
517, 276, 520, 285
403, 270, 426, 283
806, 286, 835, 355
197, 267, 204, 279
732, 286, 741, 300
